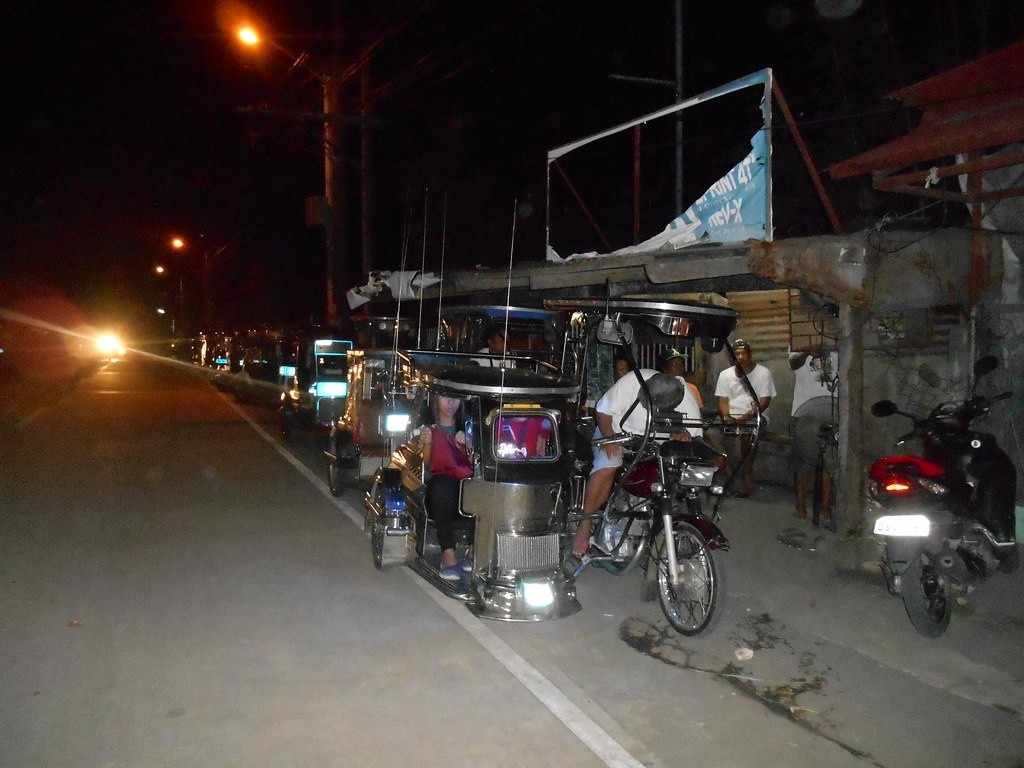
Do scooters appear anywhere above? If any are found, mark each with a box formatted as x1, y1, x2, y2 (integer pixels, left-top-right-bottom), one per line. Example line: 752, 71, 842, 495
866, 355, 1019, 639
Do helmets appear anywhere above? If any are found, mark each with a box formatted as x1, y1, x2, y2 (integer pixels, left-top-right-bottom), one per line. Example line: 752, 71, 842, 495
659, 348, 689, 365
733, 339, 751, 352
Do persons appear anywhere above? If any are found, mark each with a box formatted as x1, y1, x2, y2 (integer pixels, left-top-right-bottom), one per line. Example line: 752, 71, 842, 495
660, 345, 712, 521
471, 326, 518, 370
493, 415, 552, 458
572, 354, 704, 559
708, 339, 778, 497
418, 392, 476, 581
787, 338, 839, 526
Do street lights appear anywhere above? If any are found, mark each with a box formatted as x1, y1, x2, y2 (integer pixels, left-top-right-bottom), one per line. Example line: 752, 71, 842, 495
238, 24, 346, 339
172, 239, 214, 335
156, 266, 185, 339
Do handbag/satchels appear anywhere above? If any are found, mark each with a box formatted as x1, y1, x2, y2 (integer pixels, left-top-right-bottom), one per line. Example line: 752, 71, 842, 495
428, 424, 473, 480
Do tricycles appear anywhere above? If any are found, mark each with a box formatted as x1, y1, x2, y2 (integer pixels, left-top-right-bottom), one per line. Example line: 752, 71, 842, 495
184, 292, 763, 635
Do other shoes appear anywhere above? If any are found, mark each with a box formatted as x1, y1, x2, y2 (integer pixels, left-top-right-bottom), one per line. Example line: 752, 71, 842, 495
439, 558, 460, 581
792, 509, 807, 522
735, 491, 754, 499
573, 520, 592, 555
462, 548, 474, 571
724, 491, 732, 499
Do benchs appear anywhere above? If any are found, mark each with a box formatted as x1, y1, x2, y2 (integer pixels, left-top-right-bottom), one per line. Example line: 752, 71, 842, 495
404, 440, 475, 530
